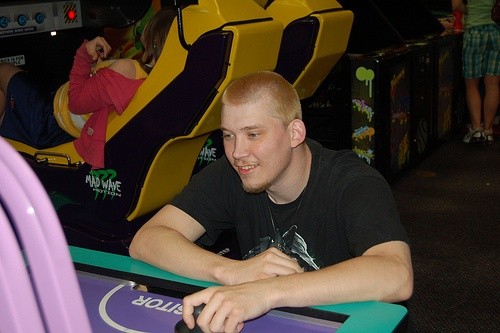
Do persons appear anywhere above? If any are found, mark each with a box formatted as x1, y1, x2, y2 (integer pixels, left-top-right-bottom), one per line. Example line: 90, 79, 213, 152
450, 0, 500, 143
129, 71, 414, 333
1, 5, 175, 170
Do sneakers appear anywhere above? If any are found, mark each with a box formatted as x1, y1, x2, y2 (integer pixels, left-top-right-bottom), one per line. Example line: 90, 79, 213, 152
485, 129, 494, 144
462, 125, 485, 145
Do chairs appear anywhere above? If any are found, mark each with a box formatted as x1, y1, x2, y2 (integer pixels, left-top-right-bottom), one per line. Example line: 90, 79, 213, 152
255, 0, 355, 116
0, 0, 283, 255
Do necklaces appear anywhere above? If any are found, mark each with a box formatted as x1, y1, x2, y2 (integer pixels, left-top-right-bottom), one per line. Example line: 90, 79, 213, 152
264, 195, 303, 253
